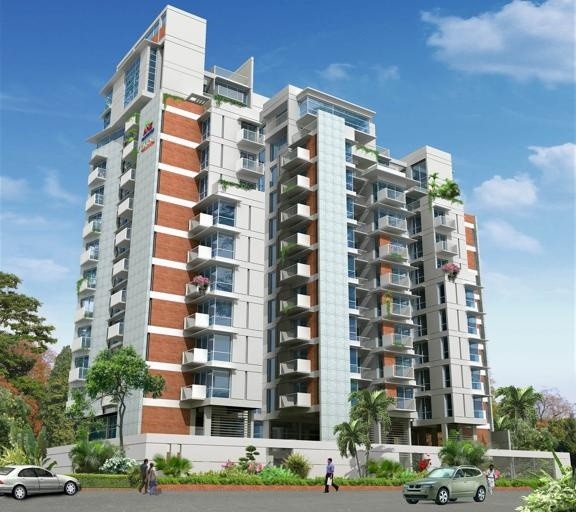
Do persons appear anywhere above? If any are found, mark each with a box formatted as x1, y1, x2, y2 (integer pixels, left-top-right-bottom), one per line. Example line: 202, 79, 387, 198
137, 458, 149, 494
482, 464, 501, 496
322, 457, 339, 494
148, 462, 157, 496
